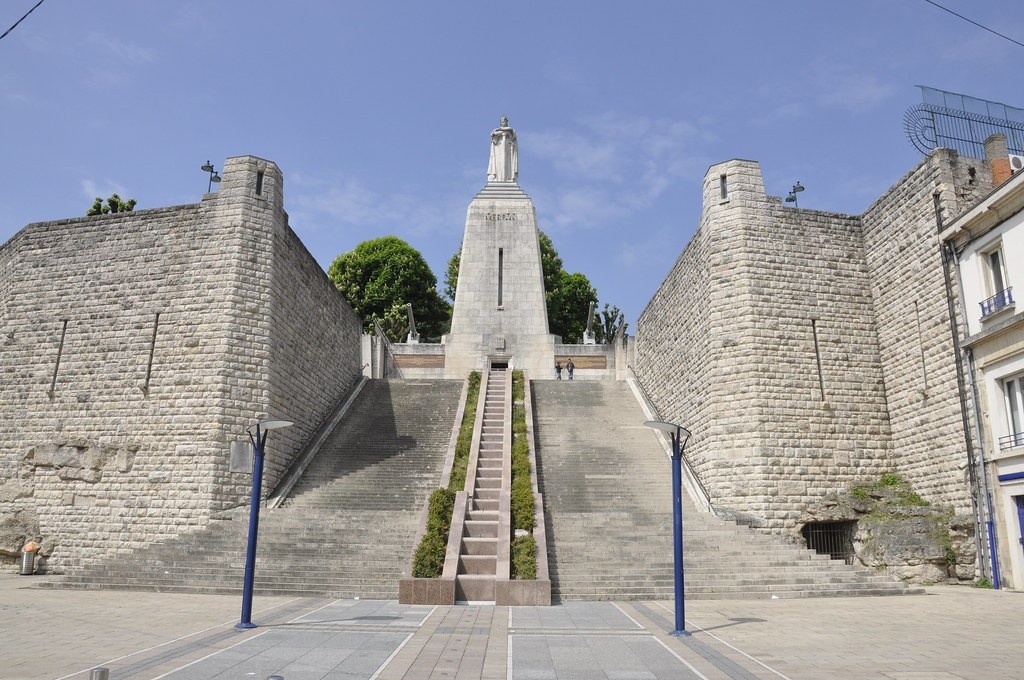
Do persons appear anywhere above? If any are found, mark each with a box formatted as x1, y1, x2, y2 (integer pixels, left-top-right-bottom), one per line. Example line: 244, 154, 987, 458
555, 362, 563, 379
566, 359, 574, 380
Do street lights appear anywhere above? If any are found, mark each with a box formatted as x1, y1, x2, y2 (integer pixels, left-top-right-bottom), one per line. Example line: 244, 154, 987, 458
201, 161, 221, 193
241, 421, 294, 623
785, 181, 805, 208
644, 421, 692, 629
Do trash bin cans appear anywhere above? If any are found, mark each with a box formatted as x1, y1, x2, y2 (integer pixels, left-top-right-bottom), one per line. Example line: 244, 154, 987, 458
19, 551, 34, 575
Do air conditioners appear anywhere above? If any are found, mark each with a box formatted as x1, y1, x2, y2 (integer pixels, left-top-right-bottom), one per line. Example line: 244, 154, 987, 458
1008, 153, 1024, 170
